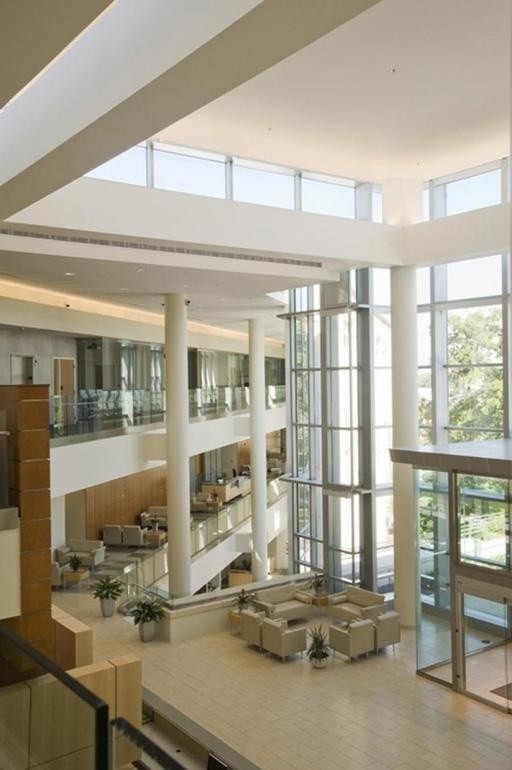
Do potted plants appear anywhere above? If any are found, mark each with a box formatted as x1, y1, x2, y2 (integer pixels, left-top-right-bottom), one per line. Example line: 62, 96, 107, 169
305, 573, 328, 594
231, 589, 254, 610
127, 597, 168, 641
303, 622, 332, 668
150, 513, 159, 531
91, 575, 125, 617
68, 553, 82, 570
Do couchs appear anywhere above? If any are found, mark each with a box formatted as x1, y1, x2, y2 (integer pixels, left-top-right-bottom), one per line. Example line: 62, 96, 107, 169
140, 505, 168, 529
253, 583, 312, 623
327, 585, 386, 623
55, 539, 106, 571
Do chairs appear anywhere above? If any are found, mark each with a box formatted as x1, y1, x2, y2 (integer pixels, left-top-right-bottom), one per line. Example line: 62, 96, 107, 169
328, 610, 401, 665
239, 609, 307, 663
191, 493, 211, 512
102, 524, 148, 550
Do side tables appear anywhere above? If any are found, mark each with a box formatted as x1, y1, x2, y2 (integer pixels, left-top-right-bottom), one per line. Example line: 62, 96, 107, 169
145, 529, 166, 547
228, 611, 242, 635
312, 595, 327, 616
61, 569, 91, 592
